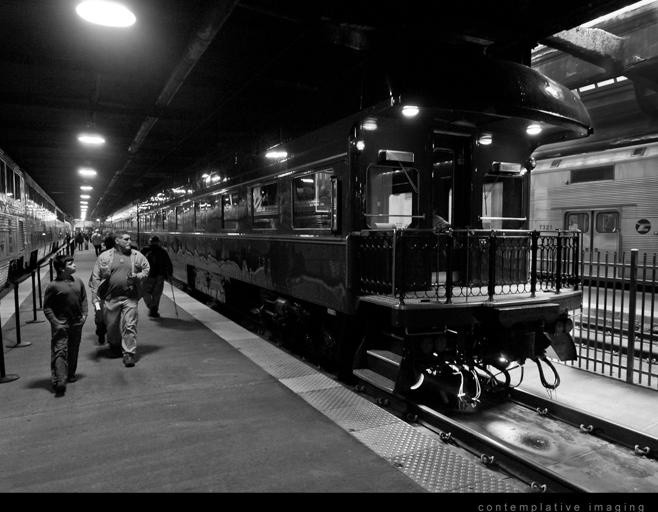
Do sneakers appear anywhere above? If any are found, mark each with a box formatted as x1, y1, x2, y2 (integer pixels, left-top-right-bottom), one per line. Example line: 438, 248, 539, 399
68, 375, 76, 381
149, 306, 159, 317
55, 382, 65, 397
110, 343, 121, 354
99, 334, 105, 343
123, 353, 135, 367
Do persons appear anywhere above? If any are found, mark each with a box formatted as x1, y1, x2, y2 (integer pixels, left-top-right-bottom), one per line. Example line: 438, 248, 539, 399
43, 255, 89, 397
91, 230, 151, 366
140, 236, 173, 318
63, 228, 115, 257
88, 274, 107, 345
432, 210, 452, 230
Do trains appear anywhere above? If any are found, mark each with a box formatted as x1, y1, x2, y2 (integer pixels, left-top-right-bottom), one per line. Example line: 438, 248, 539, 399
100, 51, 598, 423
0, 145, 74, 300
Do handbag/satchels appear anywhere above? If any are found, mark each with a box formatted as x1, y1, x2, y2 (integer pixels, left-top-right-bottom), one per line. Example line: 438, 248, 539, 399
128, 278, 141, 301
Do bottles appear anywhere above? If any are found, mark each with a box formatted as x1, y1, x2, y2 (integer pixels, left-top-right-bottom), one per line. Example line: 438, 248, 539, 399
92, 299, 102, 315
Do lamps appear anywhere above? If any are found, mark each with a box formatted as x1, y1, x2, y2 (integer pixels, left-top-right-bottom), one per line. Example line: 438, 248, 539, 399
75, 130, 107, 148
79, 183, 93, 222
75, 159, 98, 180
